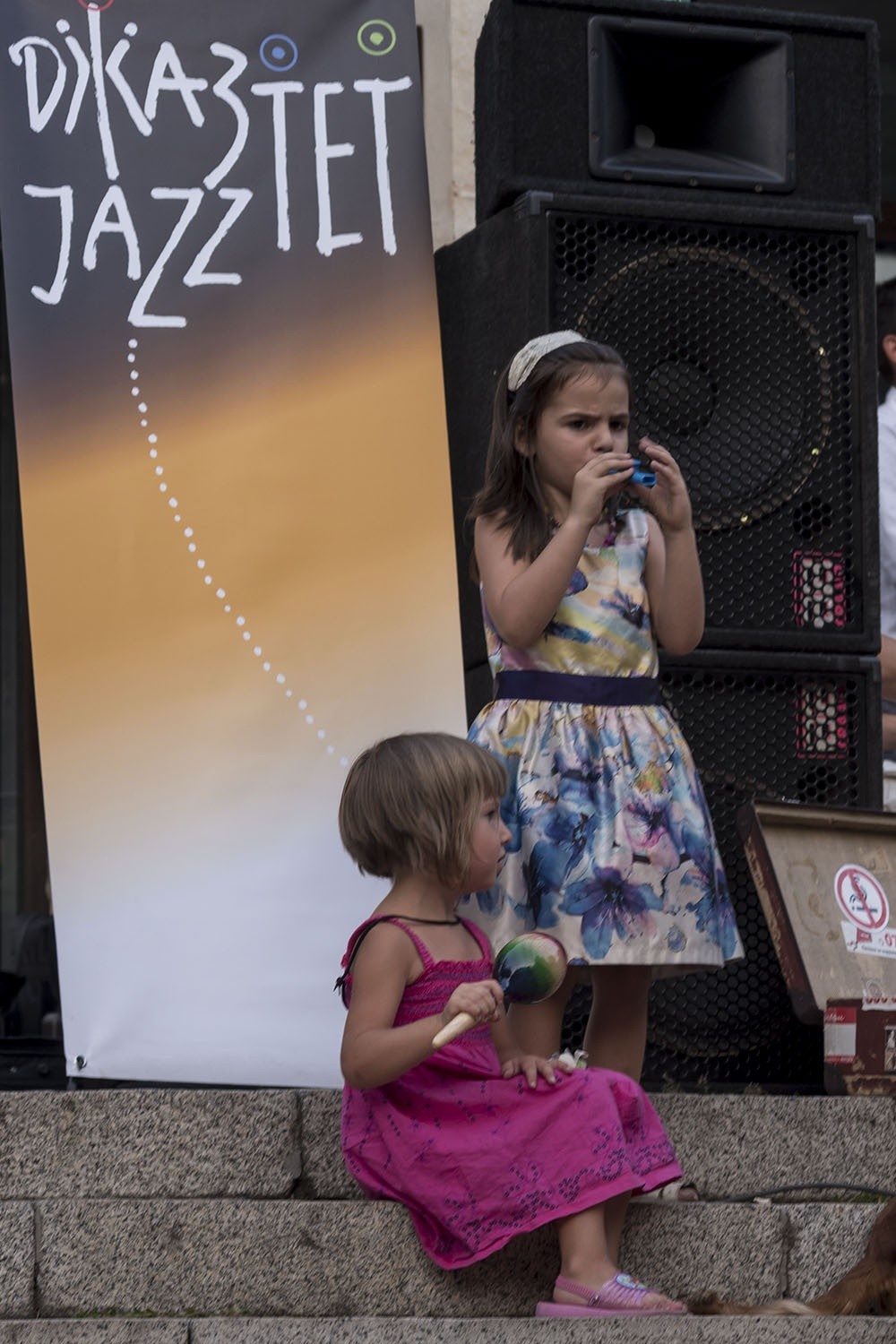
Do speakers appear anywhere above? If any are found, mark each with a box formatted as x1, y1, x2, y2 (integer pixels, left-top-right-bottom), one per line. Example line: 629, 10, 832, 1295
435, 191, 882, 1098
475, 0, 896, 243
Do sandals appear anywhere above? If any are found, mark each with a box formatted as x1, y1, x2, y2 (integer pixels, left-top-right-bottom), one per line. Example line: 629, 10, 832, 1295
629, 1181, 700, 1203
537, 1272, 687, 1316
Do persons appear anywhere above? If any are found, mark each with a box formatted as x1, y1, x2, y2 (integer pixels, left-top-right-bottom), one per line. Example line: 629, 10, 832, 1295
341, 734, 689, 1316
874, 277, 896, 751
465, 329, 742, 1090
